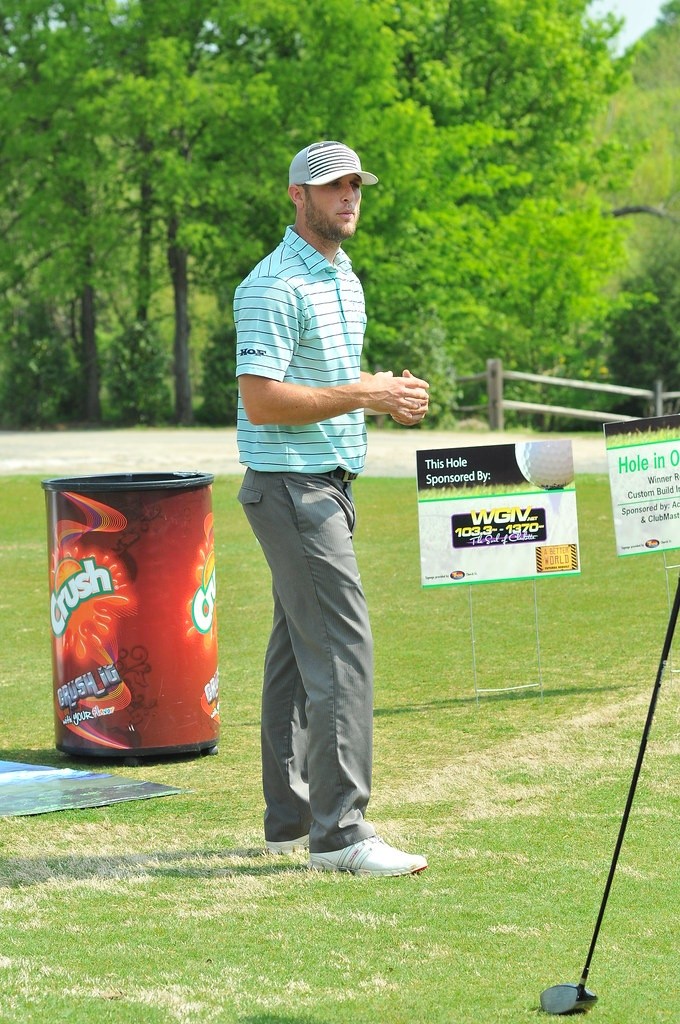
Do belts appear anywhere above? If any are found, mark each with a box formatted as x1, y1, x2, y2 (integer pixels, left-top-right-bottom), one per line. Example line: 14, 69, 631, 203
325, 469, 359, 482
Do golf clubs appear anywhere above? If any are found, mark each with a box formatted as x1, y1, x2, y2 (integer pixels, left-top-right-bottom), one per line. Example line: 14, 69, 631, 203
537, 575, 680, 1016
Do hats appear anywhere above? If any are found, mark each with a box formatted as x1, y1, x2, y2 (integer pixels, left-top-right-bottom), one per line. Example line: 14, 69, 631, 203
288, 140, 379, 185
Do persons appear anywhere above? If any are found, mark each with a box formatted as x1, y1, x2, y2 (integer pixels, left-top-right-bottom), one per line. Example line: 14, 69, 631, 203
234, 138, 429, 878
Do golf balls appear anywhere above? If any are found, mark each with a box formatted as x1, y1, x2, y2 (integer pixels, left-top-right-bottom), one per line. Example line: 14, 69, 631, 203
514, 440, 574, 489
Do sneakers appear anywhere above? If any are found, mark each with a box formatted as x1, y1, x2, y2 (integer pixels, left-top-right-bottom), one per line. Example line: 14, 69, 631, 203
264, 831, 313, 854
307, 836, 428, 877
